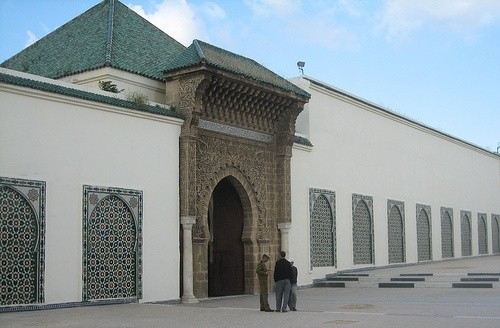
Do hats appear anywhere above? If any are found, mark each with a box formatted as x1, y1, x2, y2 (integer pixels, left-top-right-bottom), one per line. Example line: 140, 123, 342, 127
287, 258, 294, 263
264, 253, 271, 260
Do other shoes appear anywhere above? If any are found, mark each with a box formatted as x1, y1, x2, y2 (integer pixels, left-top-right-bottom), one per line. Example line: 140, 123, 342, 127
282, 310, 287, 312
275, 309, 280, 312
290, 308, 296, 311
260, 309, 265, 311
266, 309, 274, 312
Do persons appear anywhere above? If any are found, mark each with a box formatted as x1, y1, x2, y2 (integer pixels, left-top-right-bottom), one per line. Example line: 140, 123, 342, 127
288, 258, 298, 310
274, 251, 291, 312
256, 253, 274, 312
